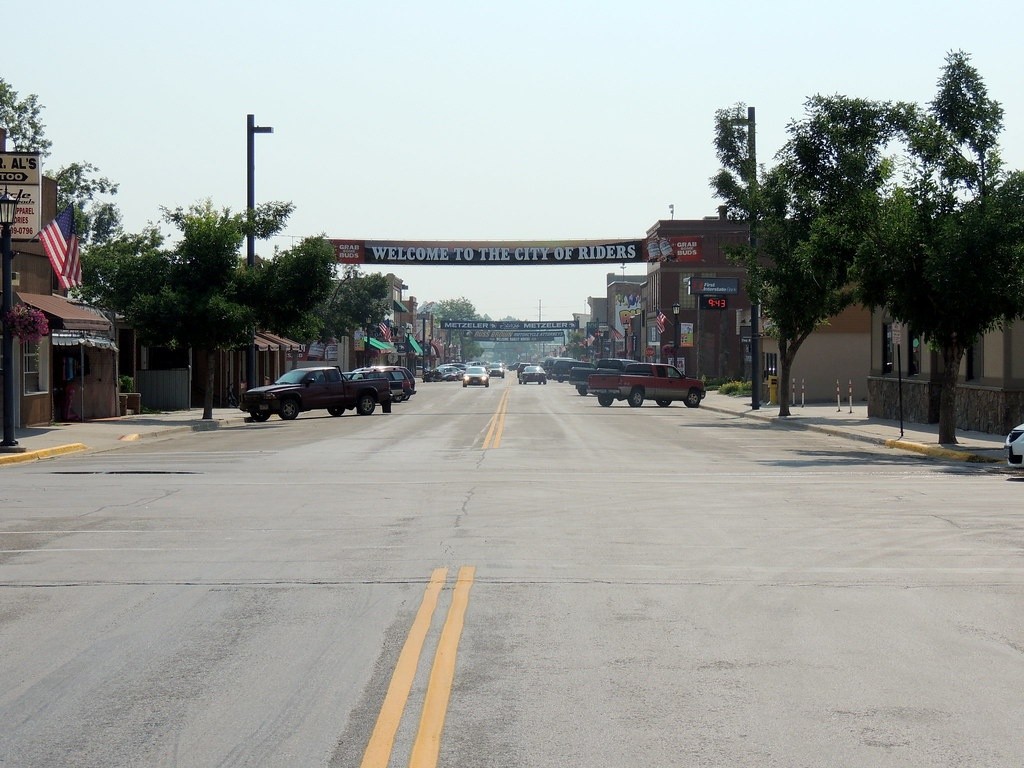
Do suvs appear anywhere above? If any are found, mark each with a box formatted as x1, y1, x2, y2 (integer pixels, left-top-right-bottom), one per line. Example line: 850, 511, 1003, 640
488, 362, 505, 378
318, 365, 418, 403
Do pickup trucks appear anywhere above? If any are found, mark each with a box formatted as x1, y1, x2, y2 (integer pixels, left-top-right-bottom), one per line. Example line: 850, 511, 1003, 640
569, 358, 643, 396
240, 365, 392, 422
586, 362, 706, 408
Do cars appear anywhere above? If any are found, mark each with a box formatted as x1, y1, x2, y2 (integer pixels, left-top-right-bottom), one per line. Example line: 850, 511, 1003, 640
461, 365, 490, 387
506, 356, 595, 383
519, 366, 547, 385
421, 360, 490, 383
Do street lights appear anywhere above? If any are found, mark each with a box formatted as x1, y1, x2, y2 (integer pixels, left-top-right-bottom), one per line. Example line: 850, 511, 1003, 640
730, 106, 761, 410
365, 314, 372, 367
0, 183, 28, 453
244, 113, 274, 391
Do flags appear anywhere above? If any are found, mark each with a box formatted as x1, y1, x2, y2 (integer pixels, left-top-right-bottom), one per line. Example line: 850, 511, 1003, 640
39, 204, 83, 289
415, 331, 422, 342
435, 338, 441, 346
379, 318, 392, 342
656, 308, 667, 334
587, 331, 595, 346
611, 326, 623, 340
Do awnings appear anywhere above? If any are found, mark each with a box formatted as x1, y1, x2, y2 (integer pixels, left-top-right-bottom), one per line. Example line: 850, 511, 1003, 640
254, 338, 268, 352
18, 292, 110, 331
363, 335, 396, 354
259, 332, 291, 351
49, 328, 119, 420
280, 336, 300, 351
255, 335, 279, 352
